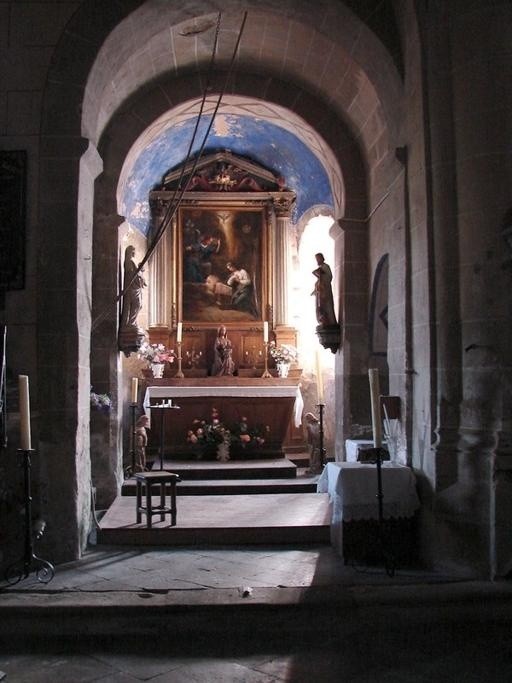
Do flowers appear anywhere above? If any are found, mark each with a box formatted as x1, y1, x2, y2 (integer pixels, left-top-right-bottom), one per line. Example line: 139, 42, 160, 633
89, 383, 113, 417
139, 341, 177, 365
272, 345, 298, 364
183, 410, 272, 461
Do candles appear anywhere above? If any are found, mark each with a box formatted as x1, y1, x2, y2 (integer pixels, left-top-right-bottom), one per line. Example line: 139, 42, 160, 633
130, 376, 140, 405
315, 350, 324, 404
176, 321, 184, 344
263, 321, 269, 343
366, 367, 385, 448
17, 372, 33, 449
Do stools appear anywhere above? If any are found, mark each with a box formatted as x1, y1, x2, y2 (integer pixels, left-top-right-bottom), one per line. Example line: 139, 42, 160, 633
135, 470, 180, 530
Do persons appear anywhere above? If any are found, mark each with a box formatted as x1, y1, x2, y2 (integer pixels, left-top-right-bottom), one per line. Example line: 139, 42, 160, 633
120, 245, 147, 328
133, 414, 150, 472
210, 325, 235, 377
310, 253, 337, 325
184, 237, 259, 319
304, 412, 325, 474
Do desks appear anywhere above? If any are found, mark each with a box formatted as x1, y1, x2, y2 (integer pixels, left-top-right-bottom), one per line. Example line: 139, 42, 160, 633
323, 460, 418, 569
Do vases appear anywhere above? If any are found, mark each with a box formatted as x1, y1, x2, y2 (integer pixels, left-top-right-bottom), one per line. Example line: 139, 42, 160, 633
150, 362, 165, 379
277, 361, 290, 379
